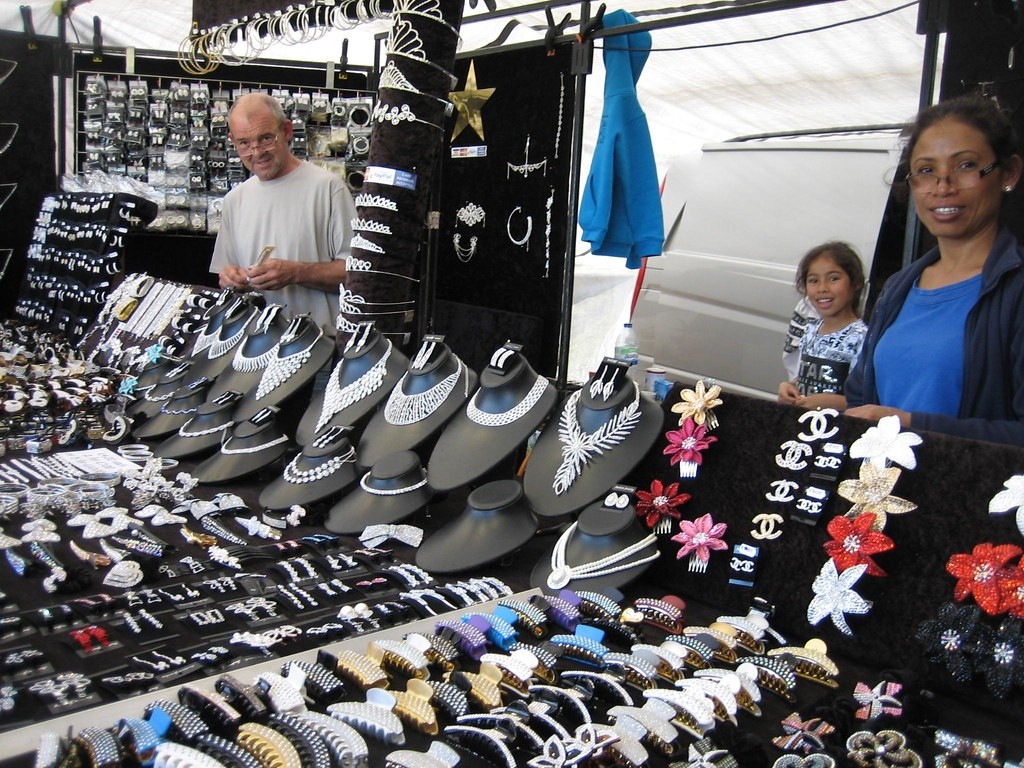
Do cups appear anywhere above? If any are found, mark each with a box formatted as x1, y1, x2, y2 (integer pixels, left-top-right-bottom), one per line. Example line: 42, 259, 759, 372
644, 368, 666, 391
641, 390, 656, 401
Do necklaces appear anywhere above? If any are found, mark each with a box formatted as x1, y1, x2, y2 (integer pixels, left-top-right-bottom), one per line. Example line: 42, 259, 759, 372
543, 187, 555, 279
554, 71, 565, 158
507, 135, 547, 178
75, 272, 660, 592
507, 206, 533, 245
452, 232, 479, 263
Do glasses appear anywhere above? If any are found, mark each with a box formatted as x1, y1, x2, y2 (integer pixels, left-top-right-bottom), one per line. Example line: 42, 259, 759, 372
234, 127, 283, 157
904, 159, 1007, 194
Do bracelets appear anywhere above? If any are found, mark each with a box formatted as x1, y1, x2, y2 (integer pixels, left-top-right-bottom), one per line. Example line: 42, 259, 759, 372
0, 442, 181, 526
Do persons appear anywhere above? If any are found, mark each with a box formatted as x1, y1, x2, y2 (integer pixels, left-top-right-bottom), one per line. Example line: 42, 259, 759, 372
207, 92, 357, 337
843, 92, 1024, 450
776, 240, 870, 412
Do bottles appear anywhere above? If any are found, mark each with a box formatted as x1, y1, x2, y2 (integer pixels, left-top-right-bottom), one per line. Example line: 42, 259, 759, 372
628, 359, 642, 391
614, 323, 639, 362
